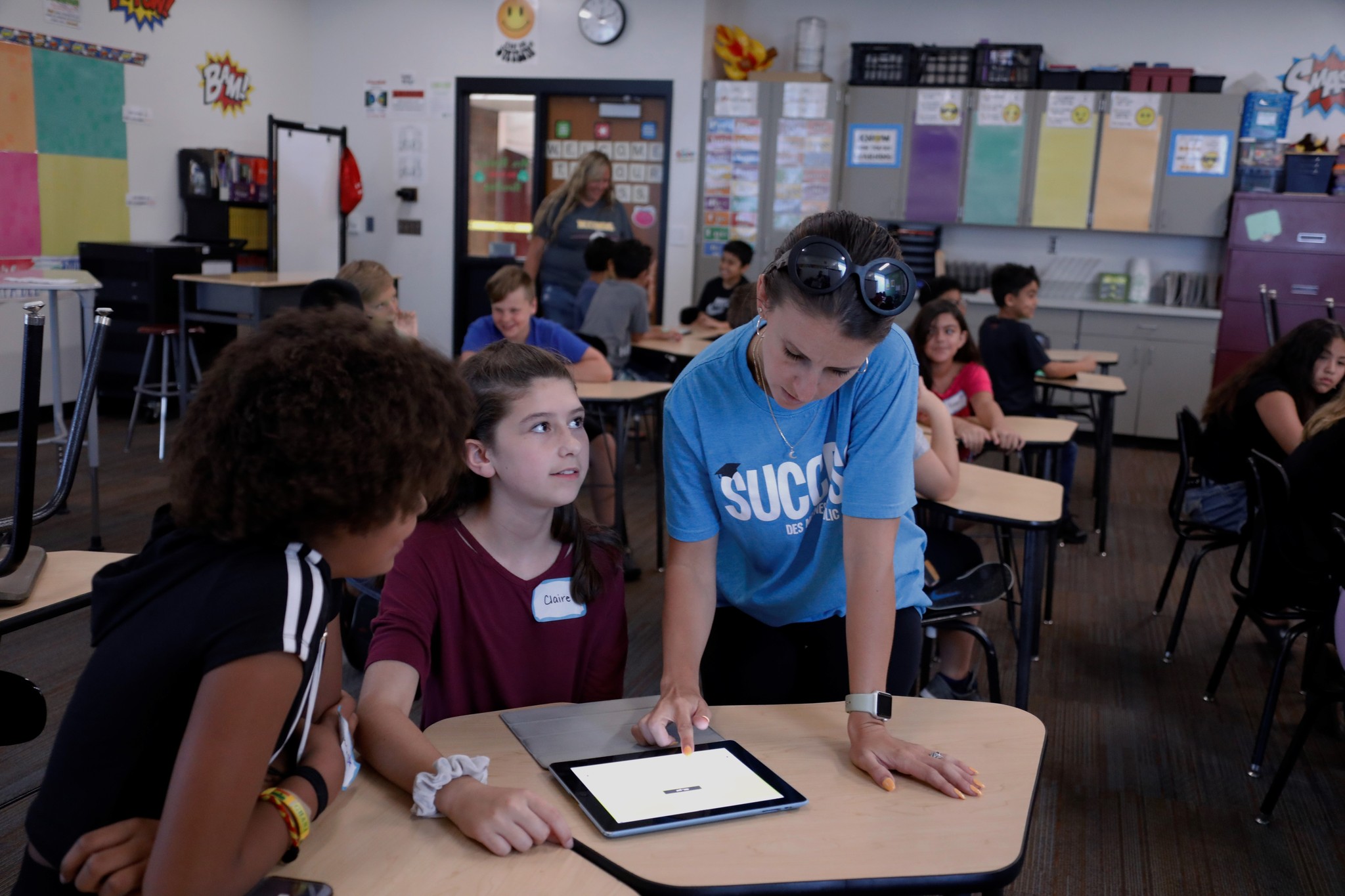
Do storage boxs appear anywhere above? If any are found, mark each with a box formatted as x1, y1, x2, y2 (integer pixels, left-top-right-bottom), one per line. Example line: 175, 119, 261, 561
974, 41, 1043, 89
846, 39, 918, 88
1241, 89, 1295, 141
1126, 66, 1197, 94
1231, 164, 1286, 195
912, 42, 978, 88
1188, 72, 1226, 94
1282, 153, 1339, 196
1236, 135, 1296, 171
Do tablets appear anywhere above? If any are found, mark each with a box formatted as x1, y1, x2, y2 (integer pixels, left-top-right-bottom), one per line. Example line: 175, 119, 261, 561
548, 740, 808, 838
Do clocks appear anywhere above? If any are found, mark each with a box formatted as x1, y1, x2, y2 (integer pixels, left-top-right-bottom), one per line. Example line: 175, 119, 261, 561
577, 0, 627, 47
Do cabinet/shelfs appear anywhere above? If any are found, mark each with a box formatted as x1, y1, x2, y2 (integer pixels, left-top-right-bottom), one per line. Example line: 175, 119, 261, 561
690, 77, 1345, 456
176, 142, 272, 276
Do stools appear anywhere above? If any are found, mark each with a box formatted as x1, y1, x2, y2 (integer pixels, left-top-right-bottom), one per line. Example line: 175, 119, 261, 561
120, 324, 206, 463
919, 599, 1005, 706
0, 294, 121, 609
947, 506, 1039, 665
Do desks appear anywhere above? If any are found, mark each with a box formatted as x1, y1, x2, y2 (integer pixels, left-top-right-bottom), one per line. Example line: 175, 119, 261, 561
630, 336, 714, 464
0, 544, 142, 896
173, 267, 333, 427
1044, 346, 1125, 512
267, 268, 406, 301
1034, 370, 1129, 558
644, 321, 732, 443
917, 413, 1081, 664
0, 268, 111, 553
915, 457, 1065, 711
574, 381, 675, 578
262, 695, 1051, 896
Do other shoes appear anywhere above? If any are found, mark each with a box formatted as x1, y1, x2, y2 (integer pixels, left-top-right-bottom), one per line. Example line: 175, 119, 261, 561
924, 559, 1015, 610
1057, 494, 1088, 545
921, 670, 982, 701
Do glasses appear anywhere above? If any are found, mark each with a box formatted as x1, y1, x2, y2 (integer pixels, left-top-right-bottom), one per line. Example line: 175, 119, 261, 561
761, 237, 917, 316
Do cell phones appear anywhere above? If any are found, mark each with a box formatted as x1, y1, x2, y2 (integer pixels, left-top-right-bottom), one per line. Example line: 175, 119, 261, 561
257, 874, 333, 896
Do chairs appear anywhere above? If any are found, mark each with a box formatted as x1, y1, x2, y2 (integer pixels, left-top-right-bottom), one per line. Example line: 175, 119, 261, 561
1196, 449, 1345, 784
1244, 492, 1345, 828
1145, 406, 1249, 667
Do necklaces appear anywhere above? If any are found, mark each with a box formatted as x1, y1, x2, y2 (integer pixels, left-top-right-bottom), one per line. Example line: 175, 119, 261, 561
757, 330, 826, 459
751, 326, 769, 389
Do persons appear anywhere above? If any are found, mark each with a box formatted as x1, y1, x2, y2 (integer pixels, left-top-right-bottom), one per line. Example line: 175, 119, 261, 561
524, 151, 634, 335
630, 210, 987, 800
457, 265, 642, 584
14, 309, 476, 896
298, 278, 365, 311
977, 263, 1096, 544
698, 239, 754, 330
917, 274, 968, 319
579, 236, 683, 415
336, 260, 416, 339
1181, 317, 1345, 667
355, 341, 628, 858
906, 298, 1027, 703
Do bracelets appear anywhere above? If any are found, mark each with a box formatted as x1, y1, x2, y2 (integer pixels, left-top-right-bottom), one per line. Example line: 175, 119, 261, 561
259, 790, 300, 866
267, 764, 285, 785
262, 786, 311, 841
410, 754, 490, 819
282, 765, 329, 821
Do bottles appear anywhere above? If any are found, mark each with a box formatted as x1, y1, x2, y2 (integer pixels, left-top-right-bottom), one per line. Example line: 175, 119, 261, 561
194, 151, 240, 201
1129, 258, 1150, 303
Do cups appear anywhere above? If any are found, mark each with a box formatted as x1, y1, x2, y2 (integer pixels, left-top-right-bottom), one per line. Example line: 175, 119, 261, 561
795, 16, 825, 72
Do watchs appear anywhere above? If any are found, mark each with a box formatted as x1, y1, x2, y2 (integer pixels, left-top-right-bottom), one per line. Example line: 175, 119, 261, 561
845, 691, 895, 721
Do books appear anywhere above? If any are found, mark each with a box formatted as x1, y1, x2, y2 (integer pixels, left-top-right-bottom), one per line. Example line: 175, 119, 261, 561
1162, 271, 1219, 308
